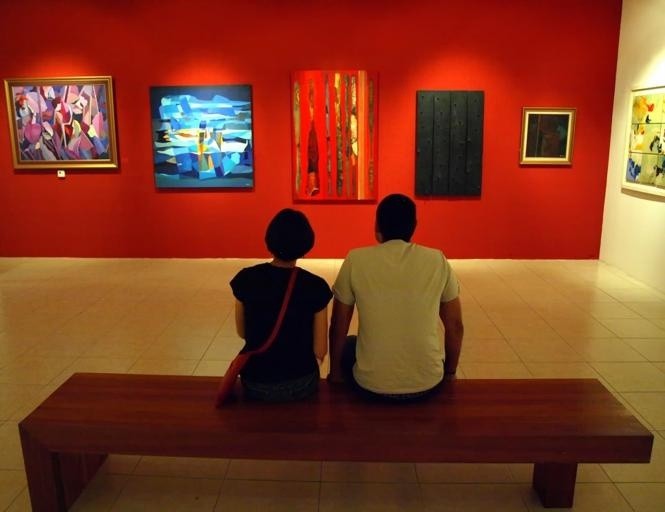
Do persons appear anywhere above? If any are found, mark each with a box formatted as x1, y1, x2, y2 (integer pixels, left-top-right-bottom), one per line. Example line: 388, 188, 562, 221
326, 193, 464, 403
228, 208, 332, 402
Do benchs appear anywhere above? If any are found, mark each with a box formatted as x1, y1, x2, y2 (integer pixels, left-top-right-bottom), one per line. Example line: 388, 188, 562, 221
14, 365, 654, 511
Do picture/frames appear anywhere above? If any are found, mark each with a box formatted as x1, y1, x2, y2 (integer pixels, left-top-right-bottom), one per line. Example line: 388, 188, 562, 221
618, 83, 665, 199
516, 103, 577, 169
0, 73, 121, 173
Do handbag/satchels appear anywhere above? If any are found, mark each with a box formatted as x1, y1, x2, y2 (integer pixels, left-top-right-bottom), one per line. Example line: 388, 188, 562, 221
212, 352, 252, 410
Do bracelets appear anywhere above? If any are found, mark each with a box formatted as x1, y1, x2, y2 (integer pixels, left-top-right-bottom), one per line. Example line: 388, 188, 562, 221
444, 369, 457, 376
